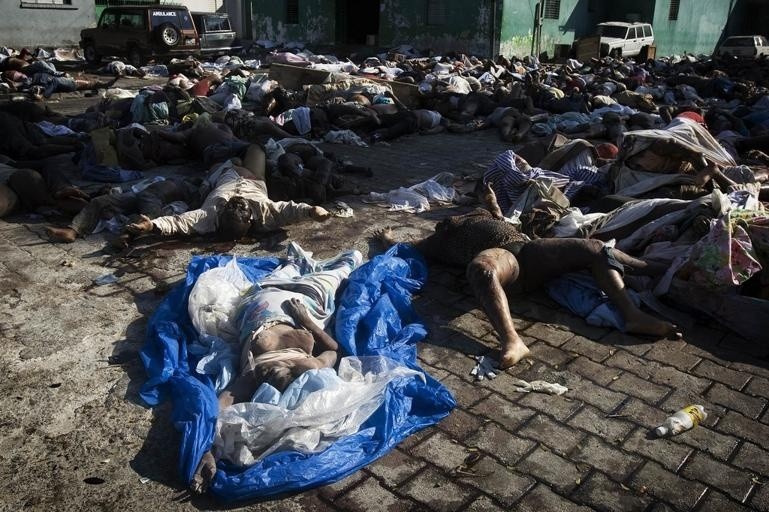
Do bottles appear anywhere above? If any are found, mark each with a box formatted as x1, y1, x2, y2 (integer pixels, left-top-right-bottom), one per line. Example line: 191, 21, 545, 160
652, 403, 709, 438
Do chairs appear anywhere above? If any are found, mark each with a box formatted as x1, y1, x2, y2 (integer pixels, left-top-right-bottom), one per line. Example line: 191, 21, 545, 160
120, 19, 133, 27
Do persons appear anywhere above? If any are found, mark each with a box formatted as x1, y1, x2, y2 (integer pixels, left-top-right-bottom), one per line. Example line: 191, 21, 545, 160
0, 47, 768, 283
369, 208, 673, 363
220, 241, 361, 407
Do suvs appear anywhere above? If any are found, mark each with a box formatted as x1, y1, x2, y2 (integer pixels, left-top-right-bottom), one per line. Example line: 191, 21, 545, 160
573, 21, 654, 58
79, 4, 200, 68
190, 12, 245, 57
715, 35, 769, 60
